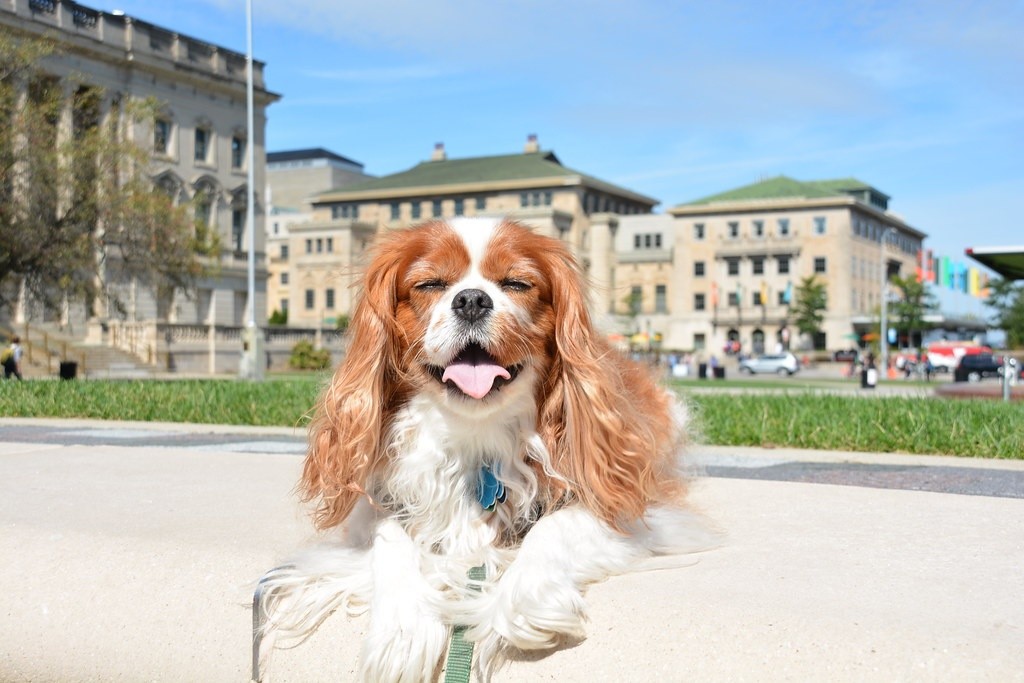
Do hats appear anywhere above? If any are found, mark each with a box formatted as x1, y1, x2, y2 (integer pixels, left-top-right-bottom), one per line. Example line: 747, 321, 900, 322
857, 340, 865, 347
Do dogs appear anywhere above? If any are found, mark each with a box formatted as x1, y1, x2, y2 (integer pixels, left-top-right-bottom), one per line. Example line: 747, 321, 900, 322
254, 216, 727, 683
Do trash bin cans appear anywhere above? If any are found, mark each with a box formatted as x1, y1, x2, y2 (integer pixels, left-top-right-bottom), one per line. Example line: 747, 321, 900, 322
60, 359, 78, 380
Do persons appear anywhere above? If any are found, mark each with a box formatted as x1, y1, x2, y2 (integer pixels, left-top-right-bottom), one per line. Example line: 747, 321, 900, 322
634, 340, 740, 372
848, 353, 931, 384
4, 335, 23, 380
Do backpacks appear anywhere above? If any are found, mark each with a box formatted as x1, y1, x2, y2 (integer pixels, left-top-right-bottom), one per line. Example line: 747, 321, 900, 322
1, 349, 14, 365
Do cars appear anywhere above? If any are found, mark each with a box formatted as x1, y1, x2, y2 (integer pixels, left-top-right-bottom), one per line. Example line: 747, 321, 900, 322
954, 352, 1024, 383
739, 349, 800, 377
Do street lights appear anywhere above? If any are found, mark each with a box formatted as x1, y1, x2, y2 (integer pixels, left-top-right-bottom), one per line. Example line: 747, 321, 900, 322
881, 224, 900, 382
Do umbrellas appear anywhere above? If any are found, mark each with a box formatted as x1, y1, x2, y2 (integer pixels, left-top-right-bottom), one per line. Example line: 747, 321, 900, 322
841, 333, 861, 350
861, 331, 880, 342
